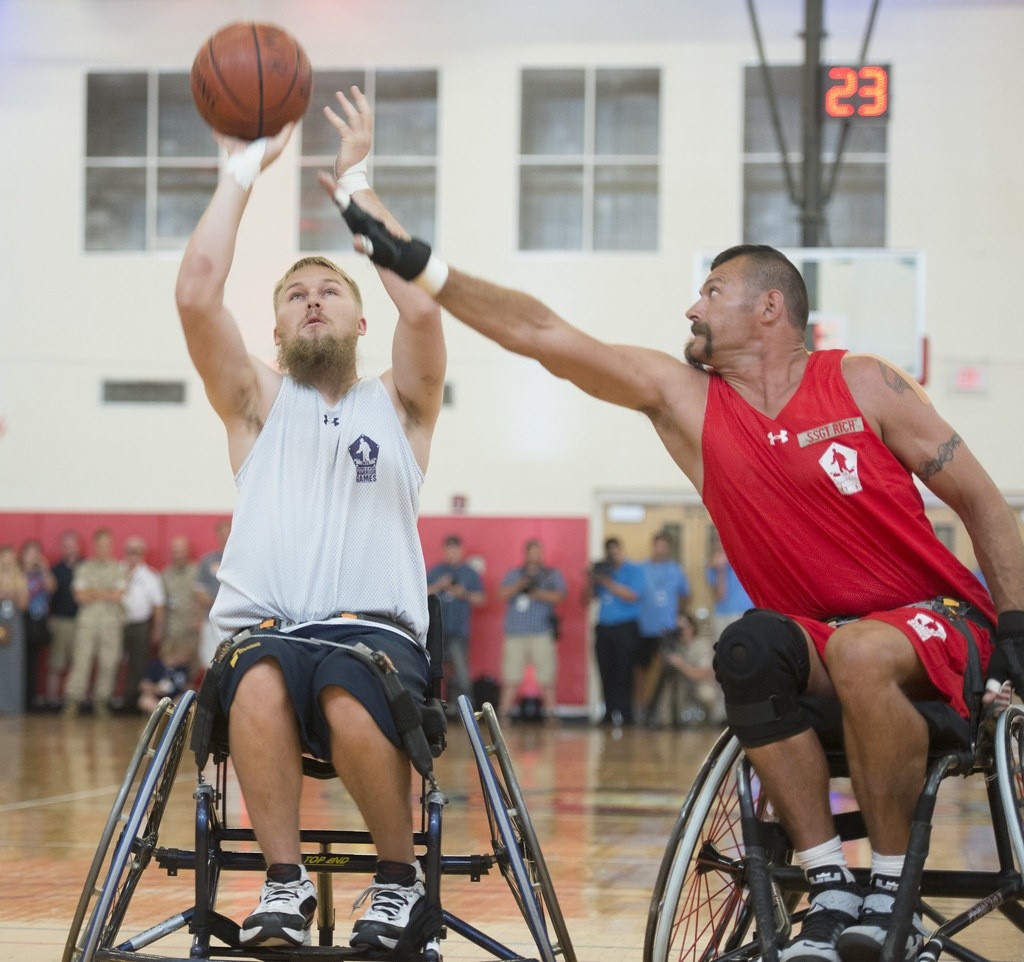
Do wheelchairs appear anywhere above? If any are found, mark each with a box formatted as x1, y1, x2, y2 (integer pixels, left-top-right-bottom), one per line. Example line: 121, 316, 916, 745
643, 662, 1024, 962
59, 591, 584, 962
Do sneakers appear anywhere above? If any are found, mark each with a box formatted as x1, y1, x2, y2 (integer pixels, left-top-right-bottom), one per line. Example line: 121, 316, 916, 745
240, 865, 317, 954
780, 865, 920, 962
346, 858, 432, 962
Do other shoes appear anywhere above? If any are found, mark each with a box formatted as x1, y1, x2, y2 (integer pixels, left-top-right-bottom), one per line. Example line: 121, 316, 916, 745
59, 699, 80, 721
93, 698, 116, 722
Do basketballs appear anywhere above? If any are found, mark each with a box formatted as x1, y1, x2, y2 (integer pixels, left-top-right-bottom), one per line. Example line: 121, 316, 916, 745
189, 20, 315, 141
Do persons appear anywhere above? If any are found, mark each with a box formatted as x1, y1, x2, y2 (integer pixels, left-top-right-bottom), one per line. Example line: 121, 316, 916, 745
0, 524, 231, 721
584, 539, 645, 726
317, 173, 1024, 962
498, 539, 565, 728
637, 531, 755, 725
174, 87, 447, 951
427, 535, 483, 709
